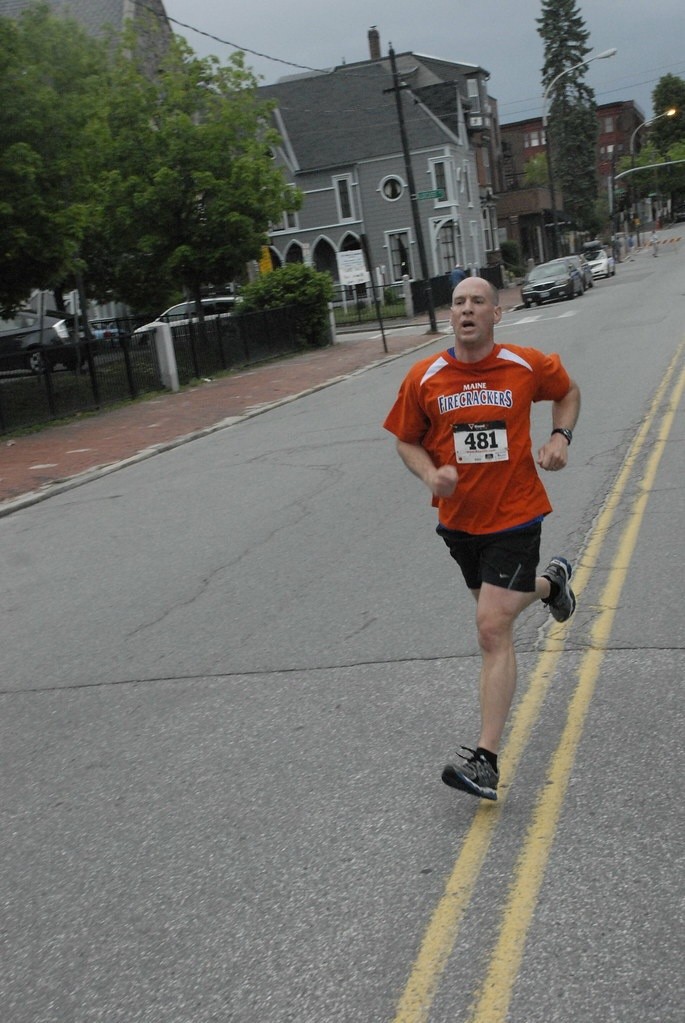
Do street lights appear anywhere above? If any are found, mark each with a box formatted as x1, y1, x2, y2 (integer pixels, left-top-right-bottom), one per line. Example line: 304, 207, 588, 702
630, 108, 675, 246
542, 48, 619, 257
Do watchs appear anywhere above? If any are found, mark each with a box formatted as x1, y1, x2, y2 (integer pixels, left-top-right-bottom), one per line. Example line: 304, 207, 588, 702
550, 428, 574, 445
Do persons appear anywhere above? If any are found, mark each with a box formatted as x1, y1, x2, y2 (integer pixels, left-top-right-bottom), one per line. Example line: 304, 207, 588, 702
384, 278, 582, 799
614, 230, 659, 263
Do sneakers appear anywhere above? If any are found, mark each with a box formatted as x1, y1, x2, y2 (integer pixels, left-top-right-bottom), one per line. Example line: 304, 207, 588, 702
441, 755, 500, 801
540, 556, 576, 624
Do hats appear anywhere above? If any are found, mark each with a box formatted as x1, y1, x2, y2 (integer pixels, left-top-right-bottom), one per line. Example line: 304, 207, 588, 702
456, 264, 463, 268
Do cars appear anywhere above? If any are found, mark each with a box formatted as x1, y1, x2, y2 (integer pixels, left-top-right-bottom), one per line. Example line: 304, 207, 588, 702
579, 240, 615, 280
517, 260, 585, 308
90, 319, 129, 347
132, 297, 245, 348
559, 256, 594, 290
0, 326, 96, 374
